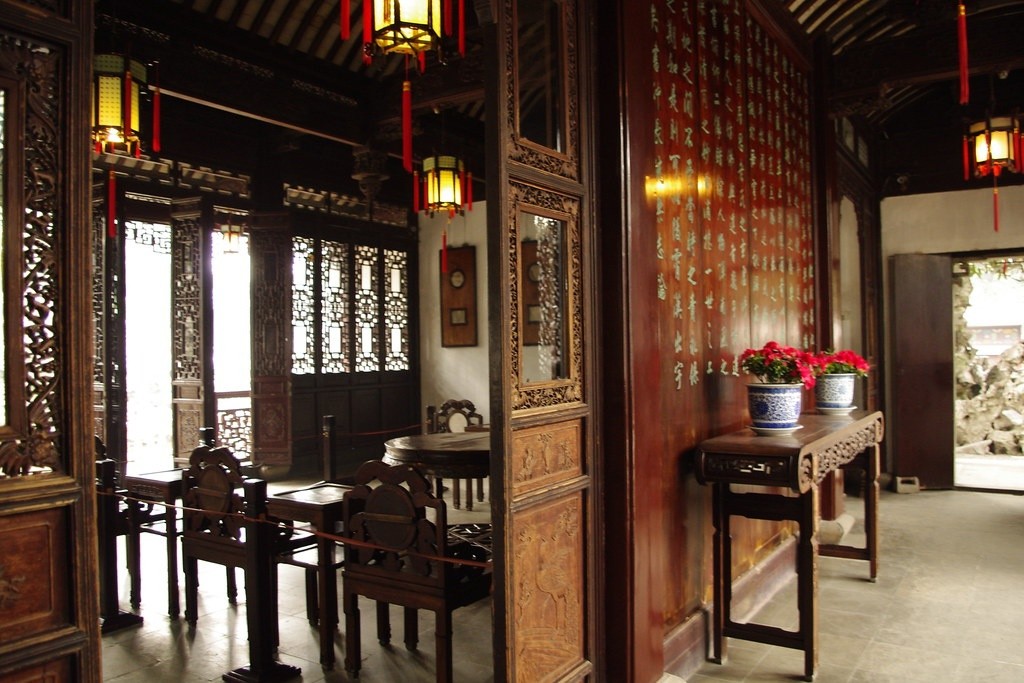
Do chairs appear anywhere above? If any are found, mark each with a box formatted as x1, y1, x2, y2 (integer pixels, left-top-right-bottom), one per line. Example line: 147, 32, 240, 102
180, 446, 294, 625
94, 433, 167, 635
341, 460, 487, 683
426, 399, 483, 511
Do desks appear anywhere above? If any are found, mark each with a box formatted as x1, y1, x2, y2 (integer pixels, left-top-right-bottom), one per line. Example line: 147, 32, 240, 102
380, 424, 492, 556
694, 409, 885, 682
124, 468, 237, 620
265, 481, 390, 675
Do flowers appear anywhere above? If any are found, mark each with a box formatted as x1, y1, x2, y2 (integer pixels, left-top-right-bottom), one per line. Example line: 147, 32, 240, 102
735, 340, 871, 392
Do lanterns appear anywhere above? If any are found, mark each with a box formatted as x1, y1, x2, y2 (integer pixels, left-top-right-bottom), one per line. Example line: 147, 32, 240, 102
413, 154, 474, 272
958, 1, 1024, 233
342, 0, 466, 174
89, 51, 161, 238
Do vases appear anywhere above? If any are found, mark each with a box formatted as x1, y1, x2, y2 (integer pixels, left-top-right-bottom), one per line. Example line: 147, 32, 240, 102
814, 373, 858, 417
745, 380, 804, 436
